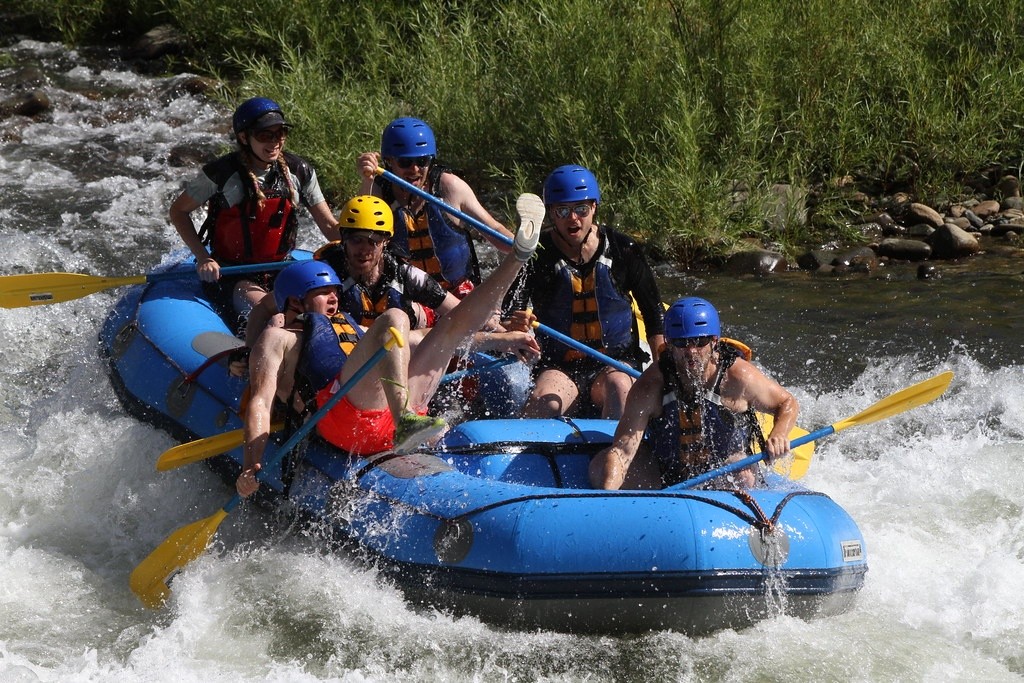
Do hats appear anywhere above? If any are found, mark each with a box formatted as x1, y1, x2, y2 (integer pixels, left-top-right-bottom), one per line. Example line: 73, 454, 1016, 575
245, 111, 293, 129
341, 228, 390, 243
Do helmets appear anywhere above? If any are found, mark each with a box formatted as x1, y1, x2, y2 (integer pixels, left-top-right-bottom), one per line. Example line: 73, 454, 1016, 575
338, 195, 394, 237
233, 97, 285, 135
273, 259, 342, 313
663, 297, 720, 341
543, 164, 601, 211
381, 117, 436, 161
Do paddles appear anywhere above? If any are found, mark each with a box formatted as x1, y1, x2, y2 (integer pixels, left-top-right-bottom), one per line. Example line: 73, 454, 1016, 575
0, 259, 330, 310
128, 326, 404, 611
365, 166, 671, 345
525, 308, 815, 480
156, 350, 529, 472
662, 372, 954, 492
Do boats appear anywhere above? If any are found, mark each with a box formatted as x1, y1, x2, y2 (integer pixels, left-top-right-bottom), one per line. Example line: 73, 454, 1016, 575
97, 245, 872, 640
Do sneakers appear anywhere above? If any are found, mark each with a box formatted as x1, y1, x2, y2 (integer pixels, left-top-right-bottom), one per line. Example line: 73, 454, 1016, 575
513, 193, 546, 261
393, 411, 448, 457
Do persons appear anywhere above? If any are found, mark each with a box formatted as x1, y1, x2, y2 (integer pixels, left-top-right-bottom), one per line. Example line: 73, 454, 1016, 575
167, 97, 342, 343
316, 192, 510, 422
236, 191, 546, 500
357, 117, 516, 329
500, 164, 668, 421
587, 297, 800, 493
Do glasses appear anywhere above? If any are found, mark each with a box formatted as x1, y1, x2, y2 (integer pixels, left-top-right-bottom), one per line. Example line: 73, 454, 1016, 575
392, 155, 434, 169
549, 202, 593, 219
247, 127, 288, 143
347, 236, 386, 247
668, 335, 713, 348
